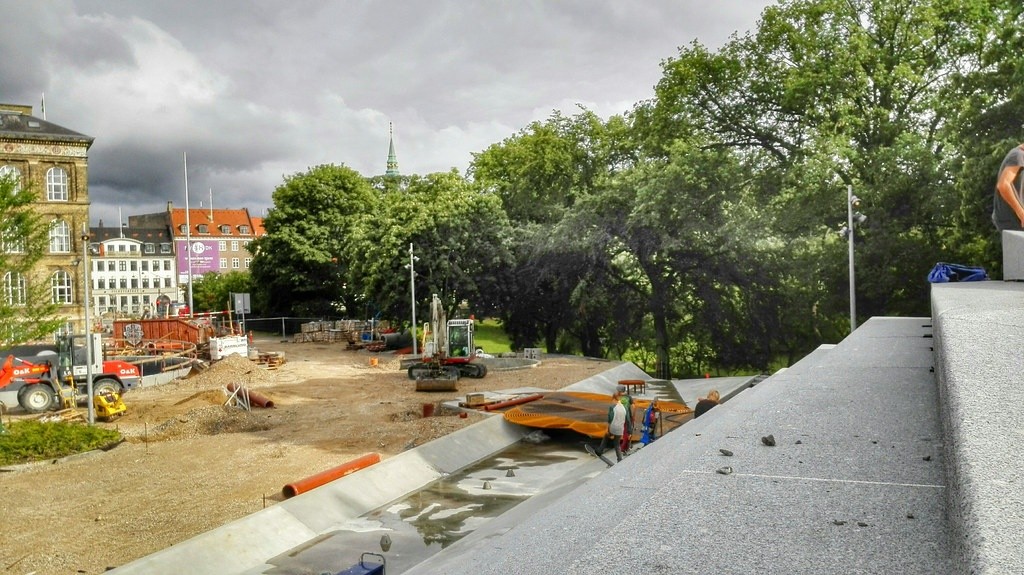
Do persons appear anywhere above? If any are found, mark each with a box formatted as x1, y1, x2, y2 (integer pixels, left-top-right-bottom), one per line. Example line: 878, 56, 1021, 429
991, 144, 1024, 234
694, 390, 720, 418
640, 396, 661, 448
592, 392, 626, 465
616, 385, 636, 453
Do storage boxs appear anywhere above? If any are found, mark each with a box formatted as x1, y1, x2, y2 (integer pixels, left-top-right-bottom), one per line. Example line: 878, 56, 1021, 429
293, 320, 390, 342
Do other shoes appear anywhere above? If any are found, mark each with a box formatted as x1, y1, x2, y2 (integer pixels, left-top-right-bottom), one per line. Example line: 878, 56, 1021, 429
593, 450, 601, 457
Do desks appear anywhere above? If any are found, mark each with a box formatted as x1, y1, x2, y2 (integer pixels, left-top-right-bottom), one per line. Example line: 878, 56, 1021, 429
618, 380, 645, 395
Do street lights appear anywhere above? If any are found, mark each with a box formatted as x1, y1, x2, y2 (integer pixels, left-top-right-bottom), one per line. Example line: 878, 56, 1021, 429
405, 249, 420, 355
837, 195, 867, 334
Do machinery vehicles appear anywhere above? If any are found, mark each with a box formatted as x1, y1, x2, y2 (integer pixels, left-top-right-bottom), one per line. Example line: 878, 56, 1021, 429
399, 315, 488, 391
0, 330, 143, 414
